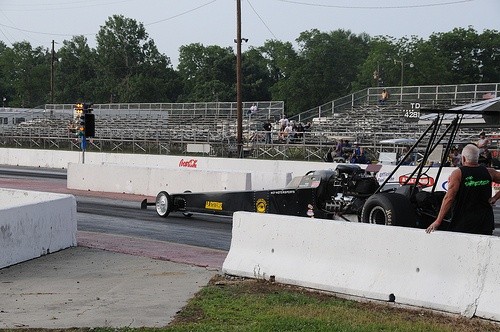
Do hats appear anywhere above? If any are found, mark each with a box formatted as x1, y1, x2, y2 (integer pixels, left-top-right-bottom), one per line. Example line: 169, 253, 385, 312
352, 142, 358, 145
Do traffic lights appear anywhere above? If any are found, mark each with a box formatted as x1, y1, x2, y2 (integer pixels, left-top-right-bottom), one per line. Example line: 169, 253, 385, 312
69, 103, 95, 138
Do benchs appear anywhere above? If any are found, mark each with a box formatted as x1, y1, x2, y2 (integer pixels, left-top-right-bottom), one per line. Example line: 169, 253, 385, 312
1, 99, 500, 148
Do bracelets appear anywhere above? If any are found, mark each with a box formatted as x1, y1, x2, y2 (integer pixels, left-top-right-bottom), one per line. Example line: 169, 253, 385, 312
435, 219, 442, 224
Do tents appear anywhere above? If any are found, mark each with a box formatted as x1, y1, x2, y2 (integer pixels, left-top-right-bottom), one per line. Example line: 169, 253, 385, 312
379, 137, 418, 167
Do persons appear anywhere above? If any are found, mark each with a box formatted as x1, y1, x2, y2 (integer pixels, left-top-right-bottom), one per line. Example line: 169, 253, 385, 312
398, 145, 412, 166
330, 137, 352, 160
246, 103, 258, 118
377, 88, 390, 105
476, 131, 492, 168
423, 143, 500, 236
448, 148, 463, 167
265, 114, 312, 144
